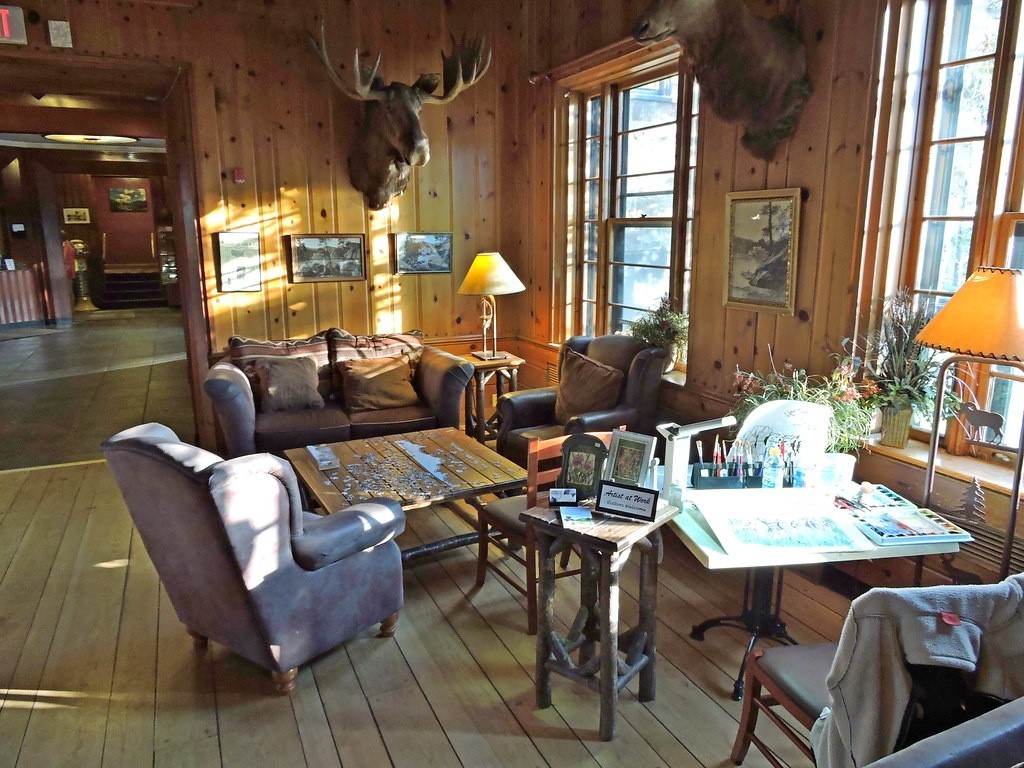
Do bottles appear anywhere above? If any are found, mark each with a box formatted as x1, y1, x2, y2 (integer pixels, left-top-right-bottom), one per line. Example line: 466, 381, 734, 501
792, 450, 809, 486
761, 448, 784, 488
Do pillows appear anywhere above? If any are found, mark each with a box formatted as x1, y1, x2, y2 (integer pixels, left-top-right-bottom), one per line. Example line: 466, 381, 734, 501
554, 347, 624, 424
227, 326, 424, 413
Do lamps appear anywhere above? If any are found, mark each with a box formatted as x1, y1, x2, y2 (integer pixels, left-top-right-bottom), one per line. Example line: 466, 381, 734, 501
656, 415, 737, 502
910, 267, 1024, 587
458, 252, 527, 359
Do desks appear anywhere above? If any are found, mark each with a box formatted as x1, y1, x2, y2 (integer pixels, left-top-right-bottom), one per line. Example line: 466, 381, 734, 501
648, 462, 960, 701
518, 495, 681, 743
459, 352, 526, 445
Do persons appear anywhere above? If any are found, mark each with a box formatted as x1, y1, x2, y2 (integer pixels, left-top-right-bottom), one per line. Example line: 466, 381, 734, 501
61, 231, 77, 315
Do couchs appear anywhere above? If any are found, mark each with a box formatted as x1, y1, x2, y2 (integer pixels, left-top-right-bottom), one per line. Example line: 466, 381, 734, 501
201, 346, 476, 457
496, 335, 668, 497
101, 422, 405, 695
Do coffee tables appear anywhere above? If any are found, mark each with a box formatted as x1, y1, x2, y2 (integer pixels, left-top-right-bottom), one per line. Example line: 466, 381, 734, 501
282, 427, 527, 560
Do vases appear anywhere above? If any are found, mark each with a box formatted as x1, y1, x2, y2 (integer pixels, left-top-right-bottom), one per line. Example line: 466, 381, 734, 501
666, 342, 676, 375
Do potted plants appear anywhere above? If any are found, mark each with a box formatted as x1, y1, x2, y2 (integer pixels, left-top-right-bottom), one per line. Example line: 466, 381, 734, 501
840, 285, 983, 454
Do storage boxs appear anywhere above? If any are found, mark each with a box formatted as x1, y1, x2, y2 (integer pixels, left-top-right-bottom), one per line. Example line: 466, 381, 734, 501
794, 452, 854, 486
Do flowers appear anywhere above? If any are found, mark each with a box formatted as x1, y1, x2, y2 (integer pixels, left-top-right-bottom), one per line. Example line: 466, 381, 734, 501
721, 343, 877, 464
631, 293, 688, 366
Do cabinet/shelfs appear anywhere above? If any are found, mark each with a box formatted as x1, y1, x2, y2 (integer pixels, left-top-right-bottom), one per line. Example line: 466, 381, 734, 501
425, 341, 1024, 601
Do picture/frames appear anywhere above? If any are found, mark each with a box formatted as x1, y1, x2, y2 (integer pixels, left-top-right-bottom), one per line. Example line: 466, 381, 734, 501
394, 232, 453, 274
721, 187, 801, 316
217, 231, 262, 293
63, 207, 90, 225
553, 435, 606, 499
109, 187, 148, 213
602, 429, 658, 488
289, 233, 368, 283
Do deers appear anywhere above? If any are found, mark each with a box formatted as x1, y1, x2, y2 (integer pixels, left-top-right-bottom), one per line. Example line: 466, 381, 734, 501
309, 14, 492, 211
631, 0, 811, 161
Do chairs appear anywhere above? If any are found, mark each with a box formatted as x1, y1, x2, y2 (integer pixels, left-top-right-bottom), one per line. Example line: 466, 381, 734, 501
477, 425, 626, 634
731, 571, 1024, 768
